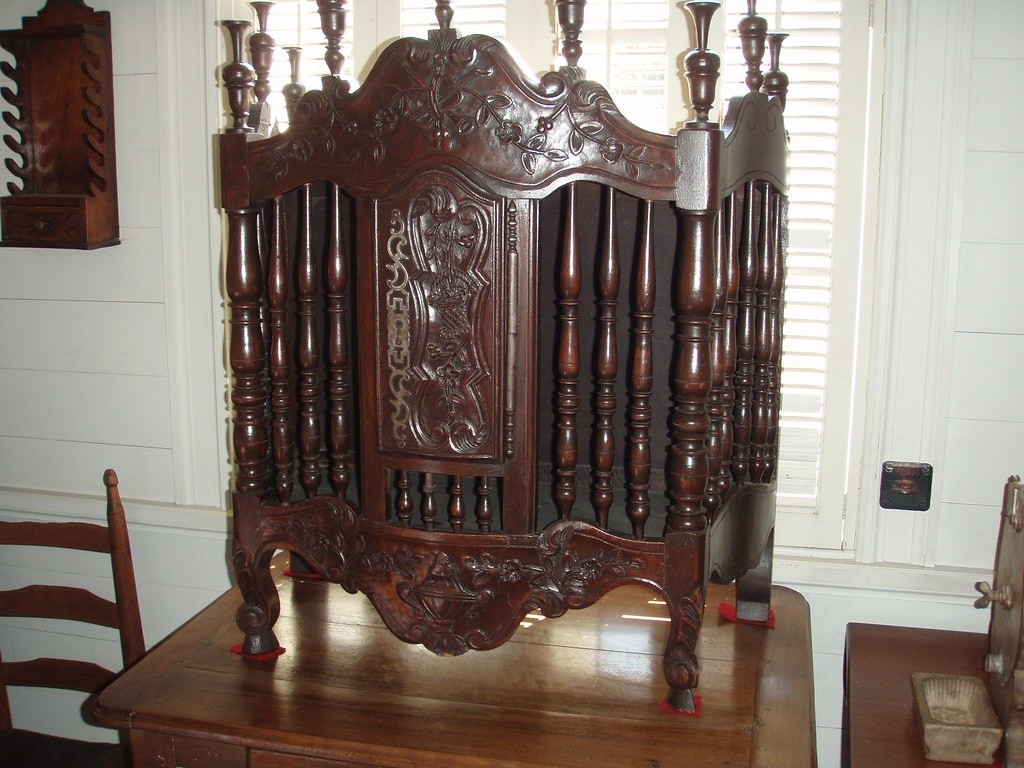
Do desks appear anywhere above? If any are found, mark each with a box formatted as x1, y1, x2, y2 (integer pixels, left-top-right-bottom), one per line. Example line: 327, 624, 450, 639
834, 617, 1006, 768
76, 547, 818, 768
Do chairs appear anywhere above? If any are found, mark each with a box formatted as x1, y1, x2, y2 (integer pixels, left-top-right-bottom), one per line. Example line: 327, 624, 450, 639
0, 466, 151, 768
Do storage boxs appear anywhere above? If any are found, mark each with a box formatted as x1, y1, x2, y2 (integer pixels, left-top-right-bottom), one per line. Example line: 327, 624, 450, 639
905, 669, 1004, 765
